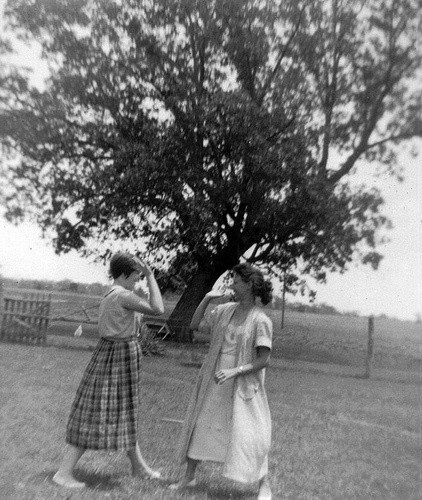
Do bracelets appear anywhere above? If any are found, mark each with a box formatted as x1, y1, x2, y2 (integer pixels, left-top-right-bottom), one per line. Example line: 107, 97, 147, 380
240, 366, 245, 375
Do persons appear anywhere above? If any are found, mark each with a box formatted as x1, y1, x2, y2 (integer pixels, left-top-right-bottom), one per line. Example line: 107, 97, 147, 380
52, 252, 164, 487
167, 264, 273, 500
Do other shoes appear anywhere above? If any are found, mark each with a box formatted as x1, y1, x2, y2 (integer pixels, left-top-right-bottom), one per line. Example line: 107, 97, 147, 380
141, 468, 160, 479
52, 470, 86, 488
257, 487, 272, 500
168, 479, 197, 490
